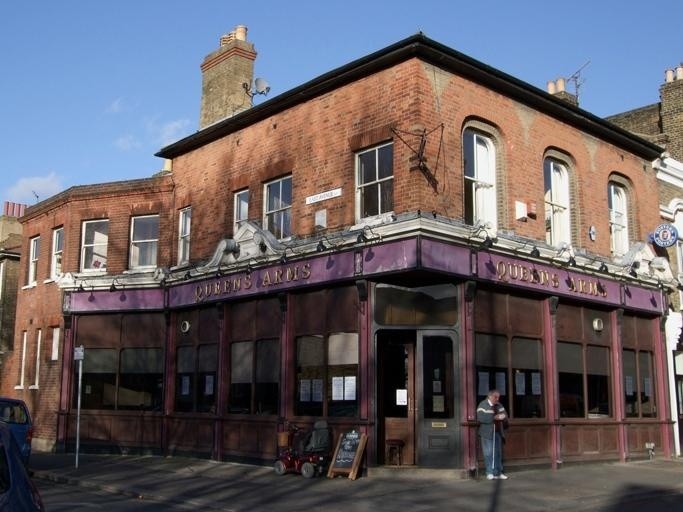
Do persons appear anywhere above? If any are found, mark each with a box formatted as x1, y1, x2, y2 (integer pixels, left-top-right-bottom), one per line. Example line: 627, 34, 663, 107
476, 389, 509, 482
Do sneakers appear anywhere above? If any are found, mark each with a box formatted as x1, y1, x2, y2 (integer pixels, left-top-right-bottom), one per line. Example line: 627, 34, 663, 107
486, 474, 494, 480
494, 475, 508, 480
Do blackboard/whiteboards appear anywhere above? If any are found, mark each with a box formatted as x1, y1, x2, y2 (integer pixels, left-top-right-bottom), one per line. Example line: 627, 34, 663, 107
330, 433, 367, 472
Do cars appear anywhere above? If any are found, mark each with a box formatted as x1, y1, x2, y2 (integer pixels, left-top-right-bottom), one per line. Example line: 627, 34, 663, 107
0, 397, 45, 512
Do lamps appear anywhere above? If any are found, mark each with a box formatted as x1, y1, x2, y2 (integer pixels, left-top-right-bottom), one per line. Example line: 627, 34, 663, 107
470, 229, 638, 280
242, 78, 270, 97
